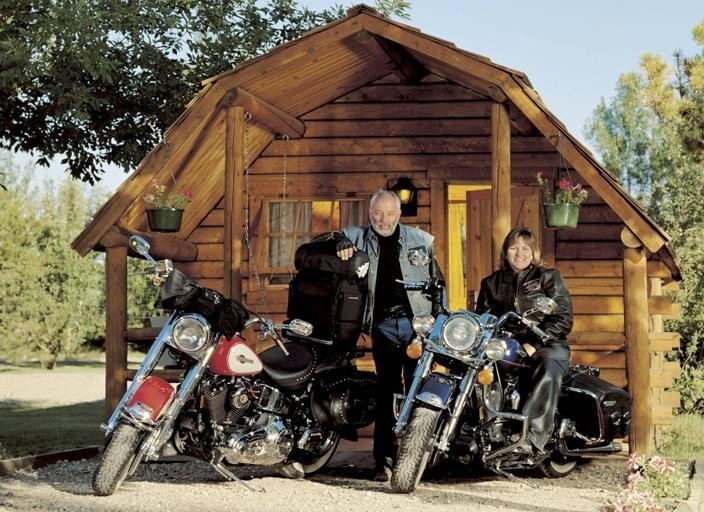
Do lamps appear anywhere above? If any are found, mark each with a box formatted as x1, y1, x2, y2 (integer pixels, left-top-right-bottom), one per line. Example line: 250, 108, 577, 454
389, 176, 418, 217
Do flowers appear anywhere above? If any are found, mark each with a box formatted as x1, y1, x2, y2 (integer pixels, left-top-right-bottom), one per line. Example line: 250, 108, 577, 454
533, 169, 588, 205
142, 179, 194, 209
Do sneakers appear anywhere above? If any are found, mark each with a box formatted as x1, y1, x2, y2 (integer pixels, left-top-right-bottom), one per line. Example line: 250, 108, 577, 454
374, 465, 393, 481
512, 434, 533, 450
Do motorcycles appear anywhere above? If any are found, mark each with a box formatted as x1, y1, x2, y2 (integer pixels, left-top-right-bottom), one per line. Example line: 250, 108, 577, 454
90, 230, 372, 499
384, 273, 635, 495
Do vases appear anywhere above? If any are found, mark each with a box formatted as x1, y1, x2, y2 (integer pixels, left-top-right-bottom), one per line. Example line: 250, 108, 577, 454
144, 209, 185, 232
544, 201, 581, 229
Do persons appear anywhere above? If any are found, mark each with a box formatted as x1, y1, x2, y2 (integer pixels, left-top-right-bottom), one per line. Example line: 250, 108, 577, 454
473, 228, 573, 455
294, 188, 445, 483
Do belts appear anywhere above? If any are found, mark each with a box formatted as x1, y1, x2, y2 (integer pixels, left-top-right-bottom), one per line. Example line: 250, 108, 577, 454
382, 305, 410, 319
